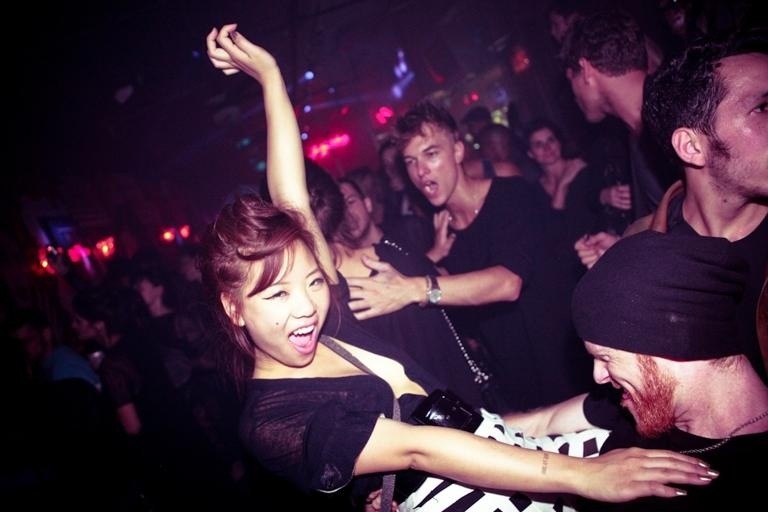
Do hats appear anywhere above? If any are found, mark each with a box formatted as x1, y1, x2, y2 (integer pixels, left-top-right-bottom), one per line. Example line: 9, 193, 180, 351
569, 228, 755, 365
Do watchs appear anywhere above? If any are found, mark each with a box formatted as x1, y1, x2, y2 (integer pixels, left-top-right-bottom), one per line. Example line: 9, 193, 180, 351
421, 273, 443, 310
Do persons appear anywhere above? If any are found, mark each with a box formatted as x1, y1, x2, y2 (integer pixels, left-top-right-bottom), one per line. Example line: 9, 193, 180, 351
562, 26, 685, 204
502, 231, 766, 512
370, 137, 436, 221
0, 256, 203, 511
206, 23, 721, 512
525, 122, 601, 268
445, 106, 539, 186
337, 178, 429, 262
573, 45, 768, 269
259, 157, 496, 412
503, 41, 551, 111
345, 100, 589, 415
